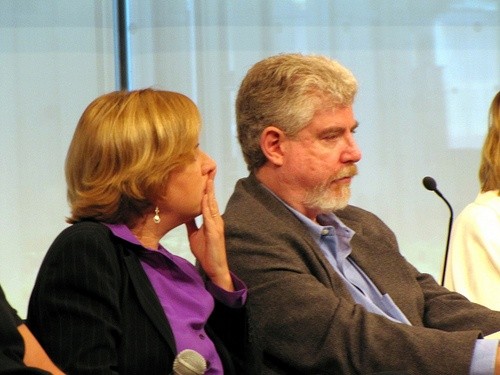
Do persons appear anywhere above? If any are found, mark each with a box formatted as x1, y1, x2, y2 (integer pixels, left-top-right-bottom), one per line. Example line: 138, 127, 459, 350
190, 53, 500, 374
0, 284, 72, 375
433, 88, 499, 339
22, 87, 267, 375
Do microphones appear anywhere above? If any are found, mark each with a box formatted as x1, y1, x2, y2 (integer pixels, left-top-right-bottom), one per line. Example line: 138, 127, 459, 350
173, 349, 207, 375
423, 176, 453, 286
